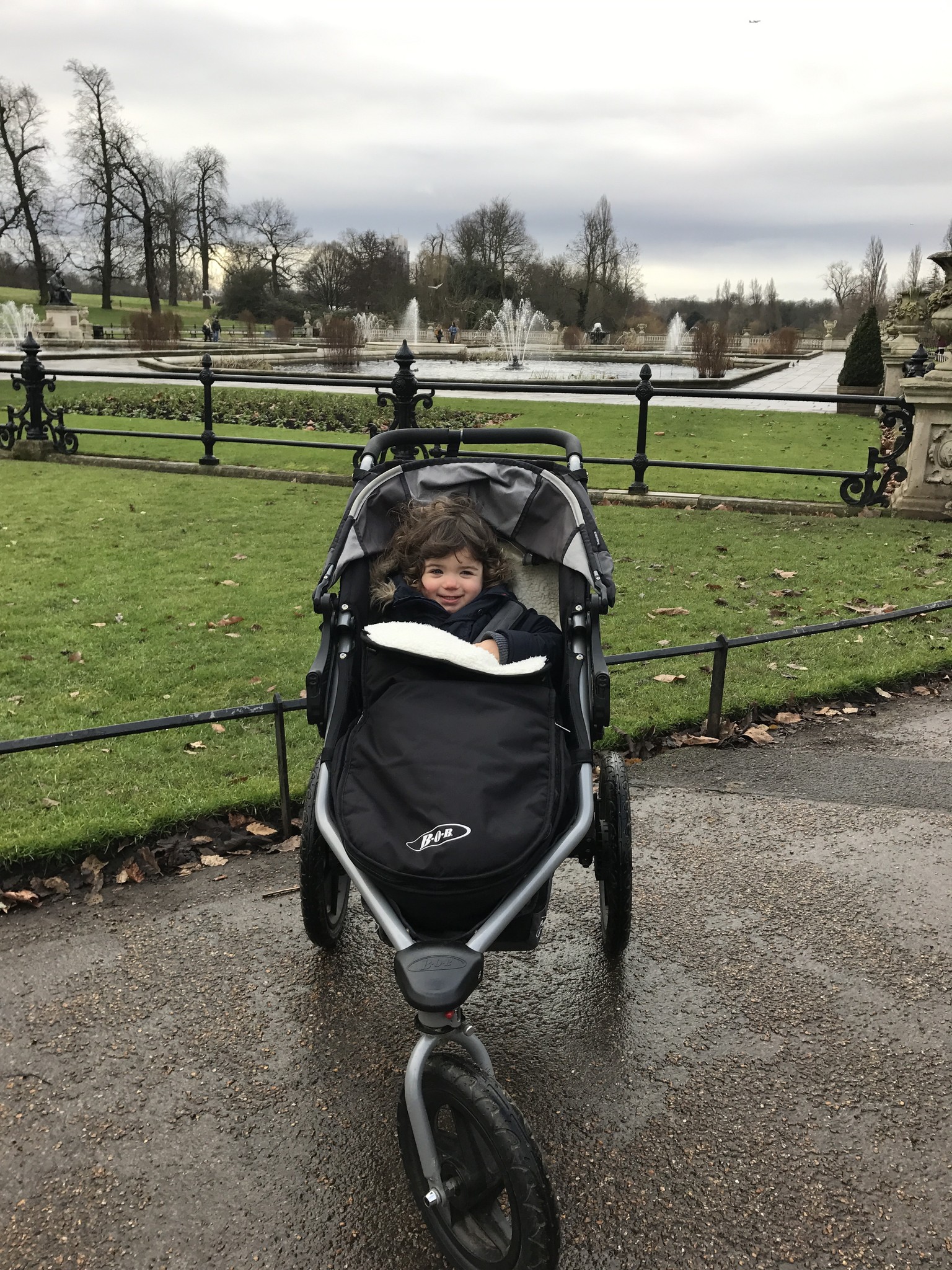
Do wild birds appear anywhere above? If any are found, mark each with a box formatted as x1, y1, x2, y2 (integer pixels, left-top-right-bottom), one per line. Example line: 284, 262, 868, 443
203, 290, 214, 301
471, 300, 479, 304
797, 359, 800, 363
428, 283, 443, 289
792, 362, 795, 367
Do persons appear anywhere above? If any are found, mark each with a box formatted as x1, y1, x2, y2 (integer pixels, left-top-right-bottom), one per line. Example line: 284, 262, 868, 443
367, 492, 564, 666
211, 317, 222, 342
435, 324, 443, 343
48, 270, 71, 303
448, 322, 457, 344
203, 319, 213, 342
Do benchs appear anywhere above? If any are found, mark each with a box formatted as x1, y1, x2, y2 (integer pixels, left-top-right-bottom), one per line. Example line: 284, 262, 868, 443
189, 332, 213, 338
5, 330, 58, 338
292, 333, 308, 338
104, 333, 131, 340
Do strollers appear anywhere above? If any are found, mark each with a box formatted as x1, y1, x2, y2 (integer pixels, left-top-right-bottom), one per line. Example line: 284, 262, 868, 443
297, 428, 633, 1269
